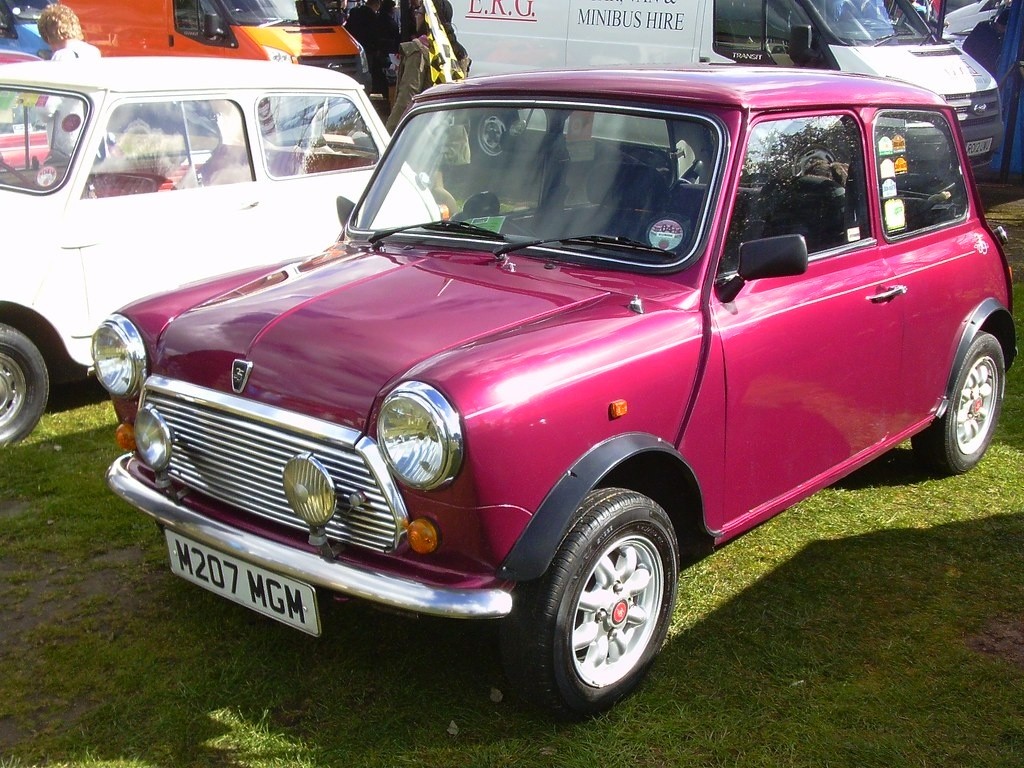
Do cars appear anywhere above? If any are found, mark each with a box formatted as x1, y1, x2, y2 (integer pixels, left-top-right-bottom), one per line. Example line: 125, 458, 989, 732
87, 64, 1016, 728
0, 58, 454, 447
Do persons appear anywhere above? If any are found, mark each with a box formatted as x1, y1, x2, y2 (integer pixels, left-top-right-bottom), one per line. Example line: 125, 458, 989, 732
34, 5, 101, 164
962, 8, 1009, 71
759, 144, 849, 256
337, 0, 471, 211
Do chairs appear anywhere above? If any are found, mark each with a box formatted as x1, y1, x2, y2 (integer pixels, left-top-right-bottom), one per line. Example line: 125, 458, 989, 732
749, 174, 847, 255
528, 163, 669, 238
90, 171, 175, 200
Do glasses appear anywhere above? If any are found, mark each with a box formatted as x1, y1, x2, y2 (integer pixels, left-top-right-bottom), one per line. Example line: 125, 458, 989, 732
414, 9, 424, 15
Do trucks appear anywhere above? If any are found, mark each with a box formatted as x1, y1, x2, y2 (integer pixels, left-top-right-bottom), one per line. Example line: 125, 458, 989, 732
447, 0, 1006, 171
59, 0, 374, 99
0, 0, 56, 59
